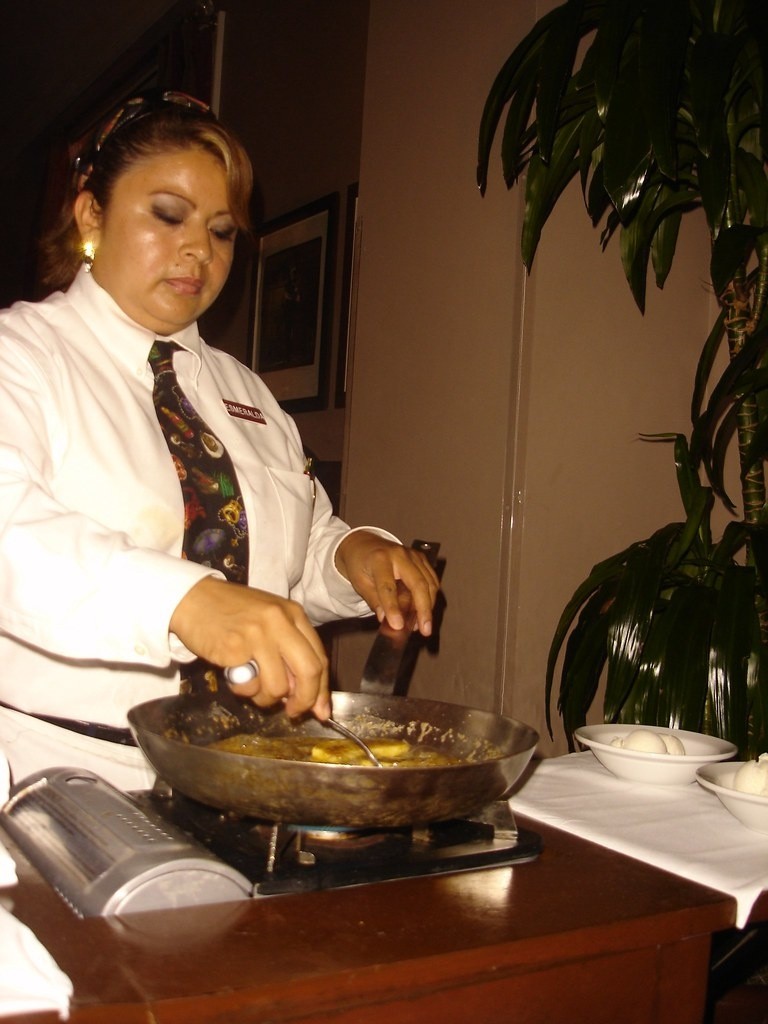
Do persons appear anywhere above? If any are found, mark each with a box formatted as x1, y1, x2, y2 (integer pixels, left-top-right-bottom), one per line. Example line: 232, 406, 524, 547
0, 92, 439, 797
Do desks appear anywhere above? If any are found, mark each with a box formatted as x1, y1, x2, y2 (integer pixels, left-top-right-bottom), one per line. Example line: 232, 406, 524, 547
502, 750, 768, 929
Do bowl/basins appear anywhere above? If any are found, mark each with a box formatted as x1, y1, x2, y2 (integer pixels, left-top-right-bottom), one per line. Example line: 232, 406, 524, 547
574, 724, 737, 786
694, 762, 768, 834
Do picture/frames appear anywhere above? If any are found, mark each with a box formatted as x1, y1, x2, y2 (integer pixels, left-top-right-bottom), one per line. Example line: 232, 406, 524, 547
334, 181, 361, 412
245, 191, 340, 413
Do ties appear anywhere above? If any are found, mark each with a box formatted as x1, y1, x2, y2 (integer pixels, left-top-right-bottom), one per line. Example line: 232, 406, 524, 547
148, 340, 249, 690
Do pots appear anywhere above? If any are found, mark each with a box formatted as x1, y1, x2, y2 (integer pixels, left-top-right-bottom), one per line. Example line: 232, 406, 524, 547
125, 538, 540, 828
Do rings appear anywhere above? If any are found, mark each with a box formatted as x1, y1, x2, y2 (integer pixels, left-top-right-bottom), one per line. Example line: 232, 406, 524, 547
224, 660, 259, 684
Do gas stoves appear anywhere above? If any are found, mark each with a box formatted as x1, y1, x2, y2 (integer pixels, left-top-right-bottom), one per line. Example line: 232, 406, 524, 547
120, 774, 544, 898
